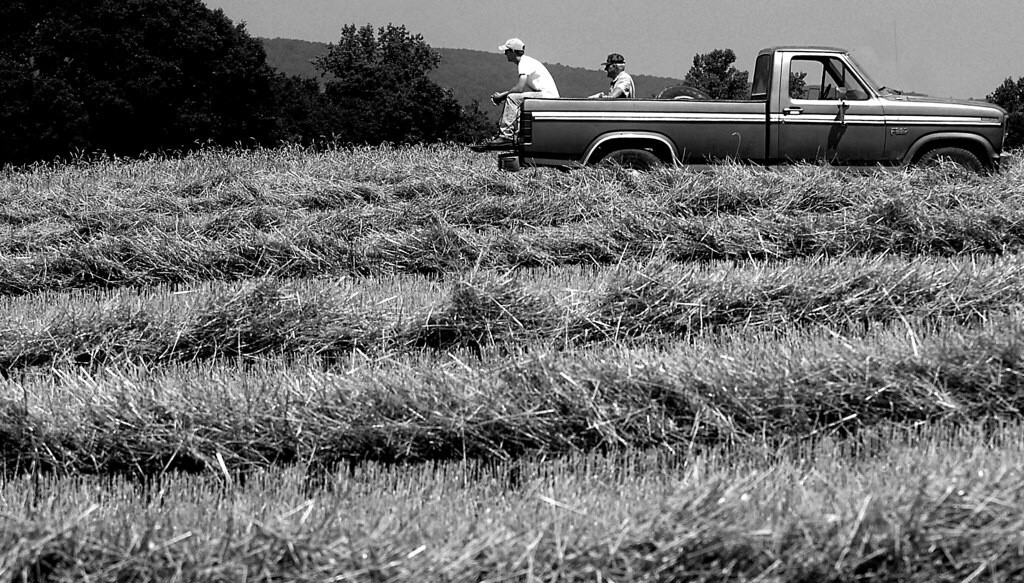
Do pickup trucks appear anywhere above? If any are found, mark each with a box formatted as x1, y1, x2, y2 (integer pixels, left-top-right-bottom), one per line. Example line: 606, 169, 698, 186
463, 44, 1012, 178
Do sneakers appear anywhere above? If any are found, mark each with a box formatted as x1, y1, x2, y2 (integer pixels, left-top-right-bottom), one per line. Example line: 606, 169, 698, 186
488, 136, 512, 143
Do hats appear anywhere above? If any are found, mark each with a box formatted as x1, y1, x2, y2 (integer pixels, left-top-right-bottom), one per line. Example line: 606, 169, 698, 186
601, 59, 625, 65
498, 37, 526, 50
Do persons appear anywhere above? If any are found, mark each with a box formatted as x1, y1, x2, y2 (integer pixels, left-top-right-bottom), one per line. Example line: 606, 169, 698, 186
486, 38, 560, 145
599, 53, 635, 99
